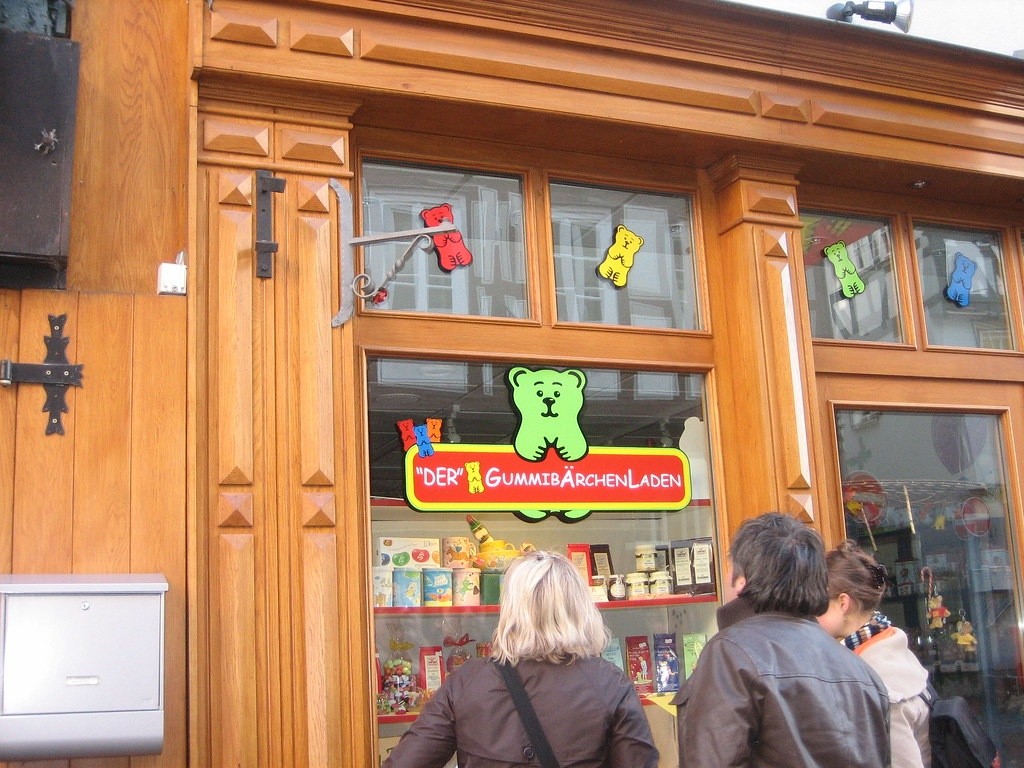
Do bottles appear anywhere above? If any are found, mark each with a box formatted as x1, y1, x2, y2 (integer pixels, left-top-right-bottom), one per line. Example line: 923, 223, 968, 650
465, 516, 493, 542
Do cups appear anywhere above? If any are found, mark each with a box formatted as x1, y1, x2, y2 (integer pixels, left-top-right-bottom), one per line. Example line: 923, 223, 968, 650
477, 538, 516, 551
424, 569, 453, 607
393, 565, 421, 607
452, 568, 481, 606
442, 537, 475, 569
372, 567, 392, 607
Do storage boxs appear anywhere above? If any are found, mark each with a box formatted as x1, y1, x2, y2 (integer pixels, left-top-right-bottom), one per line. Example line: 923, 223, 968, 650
925, 551, 945, 576
375, 536, 440, 568
826, 0, 914, 34
981, 543, 1006, 566
895, 557, 920, 587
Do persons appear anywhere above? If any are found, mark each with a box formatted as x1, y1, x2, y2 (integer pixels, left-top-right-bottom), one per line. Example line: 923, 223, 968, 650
668, 512, 893, 768
815, 536, 937, 768
379, 550, 661, 768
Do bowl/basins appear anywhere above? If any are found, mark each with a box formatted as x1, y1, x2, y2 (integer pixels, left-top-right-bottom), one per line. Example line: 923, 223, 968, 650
478, 550, 521, 565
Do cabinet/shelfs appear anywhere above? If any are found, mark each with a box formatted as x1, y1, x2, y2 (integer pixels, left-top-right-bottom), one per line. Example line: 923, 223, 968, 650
369, 498, 718, 724
858, 500, 1024, 768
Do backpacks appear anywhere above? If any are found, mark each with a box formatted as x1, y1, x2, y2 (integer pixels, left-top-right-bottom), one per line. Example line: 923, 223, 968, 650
918, 680, 1003, 768
